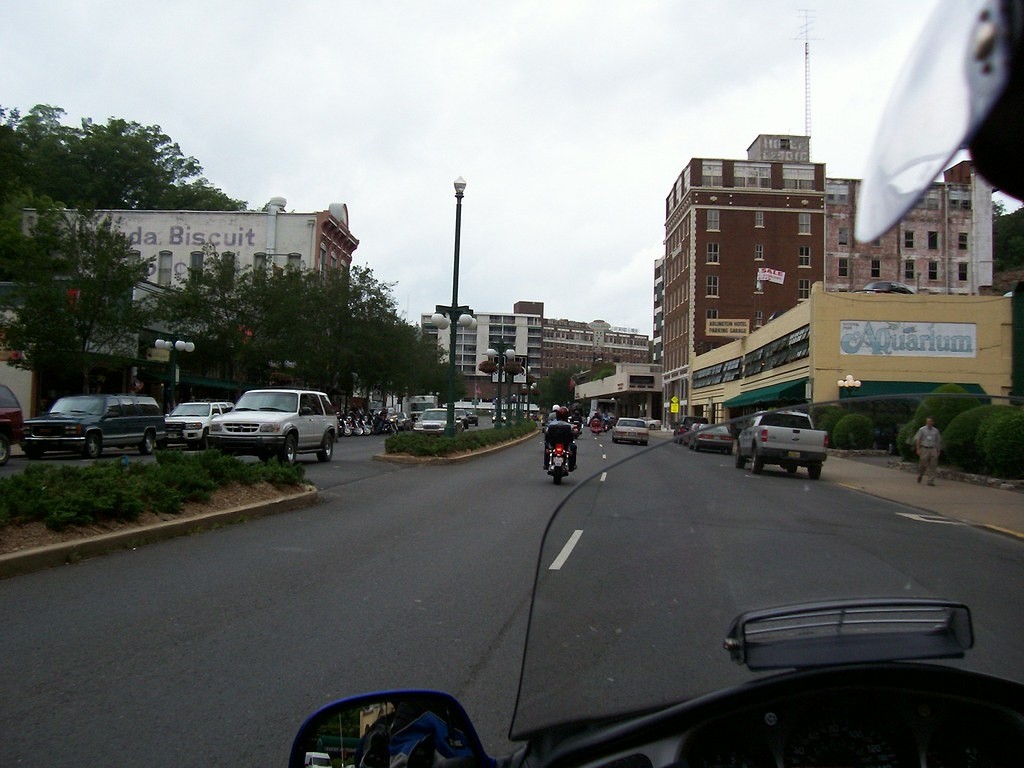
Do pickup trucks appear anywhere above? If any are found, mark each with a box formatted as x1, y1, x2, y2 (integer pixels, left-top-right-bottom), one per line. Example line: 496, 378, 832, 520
736, 410, 828, 480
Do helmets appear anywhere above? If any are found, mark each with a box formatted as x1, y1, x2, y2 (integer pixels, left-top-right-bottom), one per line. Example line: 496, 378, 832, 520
574, 410, 579, 415
556, 408, 568, 419
552, 404, 560, 411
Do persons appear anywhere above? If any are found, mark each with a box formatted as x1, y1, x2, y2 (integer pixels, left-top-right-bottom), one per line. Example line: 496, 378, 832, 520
542, 405, 582, 472
95, 375, 106, 394
913, 415, 940, 486
374, 409, 387, 435
602, 415, 613, 429
590, 413, 601, 423
546, 404, 560, 421
572, 410, 582, 435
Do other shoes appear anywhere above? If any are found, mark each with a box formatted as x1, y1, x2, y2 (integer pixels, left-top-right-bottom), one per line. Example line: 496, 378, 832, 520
917, 475, 922, 483
543, 465, 548, 470
928, 483, 936, 486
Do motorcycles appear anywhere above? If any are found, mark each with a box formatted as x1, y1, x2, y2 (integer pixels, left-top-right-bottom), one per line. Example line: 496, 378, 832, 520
542, 413, 578, 485
335, 409, 398, 437
570, 416, 584, 438
591, 420, 603, 434
604, 418, 615, 430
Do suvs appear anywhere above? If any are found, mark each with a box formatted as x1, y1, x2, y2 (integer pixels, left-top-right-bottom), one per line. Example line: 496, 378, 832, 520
206, 389, 339, 465
164, 401, 235, 451
0, 383, 167, 465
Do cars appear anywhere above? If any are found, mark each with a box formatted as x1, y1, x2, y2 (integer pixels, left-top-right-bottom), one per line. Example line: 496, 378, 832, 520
411, 408, 478, 435
491, 412, 507, 424
670, 416, 733, 454
393, 413, 413, 430
612, 417, 661, 445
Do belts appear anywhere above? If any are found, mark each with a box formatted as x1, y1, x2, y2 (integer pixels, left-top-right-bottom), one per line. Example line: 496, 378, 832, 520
922, 446, 934, 448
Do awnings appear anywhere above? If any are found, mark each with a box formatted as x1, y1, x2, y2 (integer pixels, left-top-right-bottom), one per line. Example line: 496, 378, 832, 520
149, 373, 239, 390
723, 376, 810, 408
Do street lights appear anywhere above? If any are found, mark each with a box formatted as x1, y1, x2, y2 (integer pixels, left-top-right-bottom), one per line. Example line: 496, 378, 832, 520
838, 375, 860, 413
485, 337, 515, 428
522, 382, 536, 421
155, 338, 196, 406
432, 175, 478, 437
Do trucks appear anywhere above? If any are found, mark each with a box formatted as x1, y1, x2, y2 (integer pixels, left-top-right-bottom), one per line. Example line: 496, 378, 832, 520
409, 396, 438, 423
